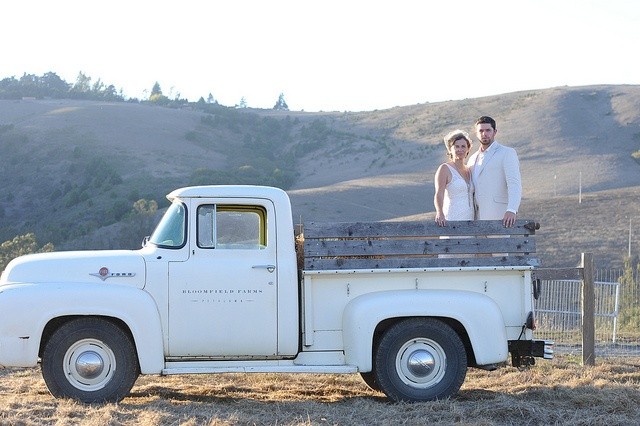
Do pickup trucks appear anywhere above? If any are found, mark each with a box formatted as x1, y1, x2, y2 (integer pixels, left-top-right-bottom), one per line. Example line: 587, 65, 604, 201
0, 185, 554, 405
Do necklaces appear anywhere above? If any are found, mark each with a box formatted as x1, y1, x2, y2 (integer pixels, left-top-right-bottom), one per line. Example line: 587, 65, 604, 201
454, 161, 467, 177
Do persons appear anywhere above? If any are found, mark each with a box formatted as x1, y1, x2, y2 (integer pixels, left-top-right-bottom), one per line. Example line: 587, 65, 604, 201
434, 129, 474, 227
467, 115, 522, 228
